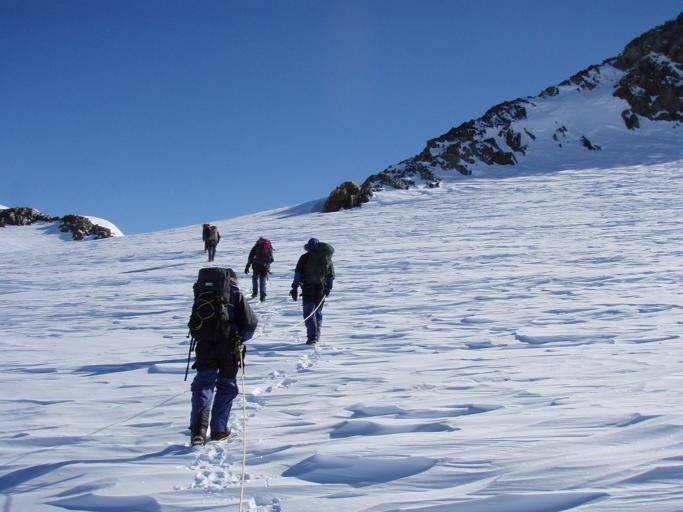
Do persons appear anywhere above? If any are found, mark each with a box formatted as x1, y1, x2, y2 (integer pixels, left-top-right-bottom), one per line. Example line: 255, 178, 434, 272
244, 237, 274, 302
202, 223, 210, 253
188, 268, 259, 446
290, 237, 336, 344
207, 226, 219, 261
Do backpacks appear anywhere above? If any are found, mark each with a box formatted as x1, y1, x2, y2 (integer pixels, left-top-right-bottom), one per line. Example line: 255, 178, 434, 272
302, 242, 334, 285
257, 240, 274, 264
189, 267, 237, 342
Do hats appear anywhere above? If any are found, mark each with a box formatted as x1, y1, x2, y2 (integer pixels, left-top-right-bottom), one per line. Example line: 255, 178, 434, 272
304, 238, 319, 251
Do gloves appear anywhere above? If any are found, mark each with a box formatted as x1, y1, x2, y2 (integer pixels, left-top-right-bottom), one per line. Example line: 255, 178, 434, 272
291, 285, 298, 300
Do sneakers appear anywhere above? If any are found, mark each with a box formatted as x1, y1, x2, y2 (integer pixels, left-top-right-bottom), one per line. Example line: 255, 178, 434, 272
252, 292, 257, 296
260, 294, 265, 300
306, 336, 317, 344
212, 426, 232, 440
191, 433, 204, 445
